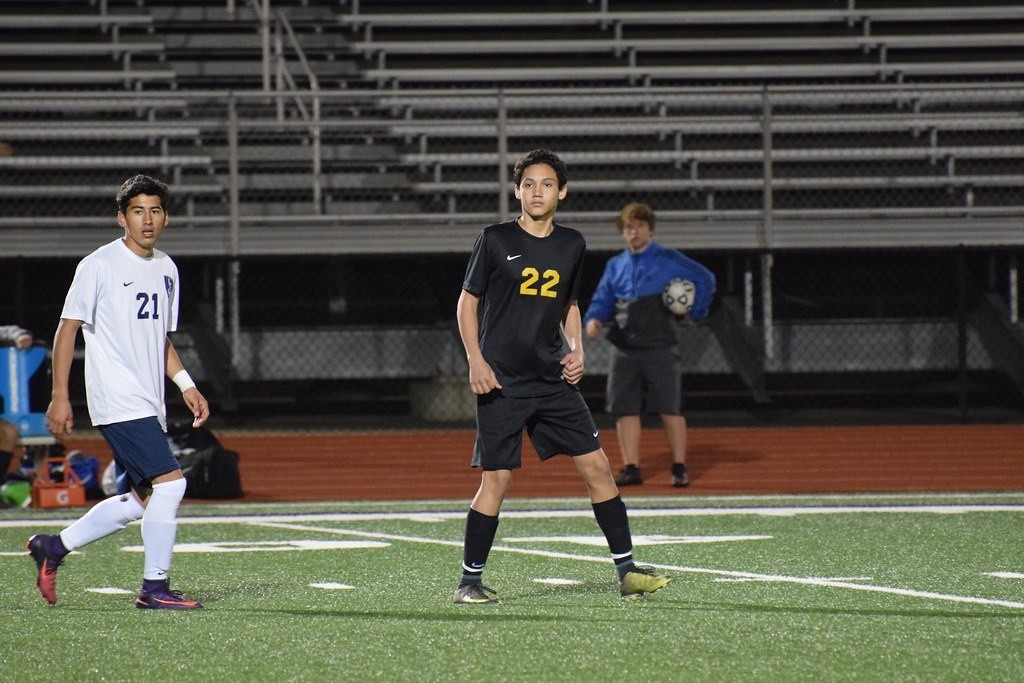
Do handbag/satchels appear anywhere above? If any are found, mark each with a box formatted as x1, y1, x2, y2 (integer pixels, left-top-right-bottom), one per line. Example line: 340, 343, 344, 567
175, 447, 245, 499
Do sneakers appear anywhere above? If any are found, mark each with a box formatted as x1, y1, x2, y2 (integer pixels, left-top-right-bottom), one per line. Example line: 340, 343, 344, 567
453, 584, 496, 604
671, 462, 688, 486
620, 570, 673, 596
135, 583, 201, 609
27, 534, 64, 604
616, 464, 642, 486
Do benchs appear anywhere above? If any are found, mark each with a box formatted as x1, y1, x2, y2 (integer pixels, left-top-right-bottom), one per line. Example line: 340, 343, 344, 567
0, 0, 1024, 220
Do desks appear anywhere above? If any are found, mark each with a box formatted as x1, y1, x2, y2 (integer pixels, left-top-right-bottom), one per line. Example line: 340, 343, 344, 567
16, 436, 57, 457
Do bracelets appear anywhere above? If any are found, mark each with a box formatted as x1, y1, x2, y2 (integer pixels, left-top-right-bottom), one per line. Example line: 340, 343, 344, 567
171, 368, 197, 392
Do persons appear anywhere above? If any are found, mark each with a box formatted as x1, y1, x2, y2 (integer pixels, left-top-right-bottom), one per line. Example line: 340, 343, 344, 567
25, 174, 209, 610
584, 202, 716, 487
455, 148, 671, 605
0, 325, 34, 512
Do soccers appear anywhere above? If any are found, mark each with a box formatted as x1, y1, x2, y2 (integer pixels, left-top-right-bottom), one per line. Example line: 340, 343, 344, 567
663, 278, 696, 313
